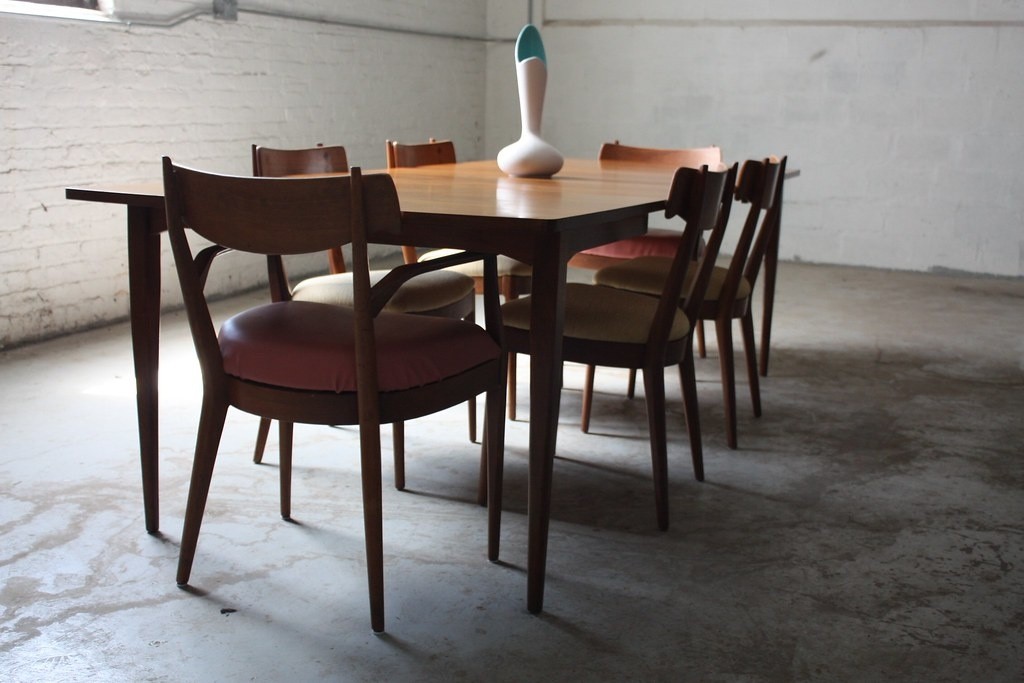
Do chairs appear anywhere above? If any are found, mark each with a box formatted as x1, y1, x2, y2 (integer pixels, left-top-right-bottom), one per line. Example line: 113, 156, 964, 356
163, 154, 507, 633
254, 136, 789, 530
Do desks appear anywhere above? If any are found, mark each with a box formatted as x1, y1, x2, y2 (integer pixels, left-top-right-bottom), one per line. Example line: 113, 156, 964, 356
65, 157, 800, 611
